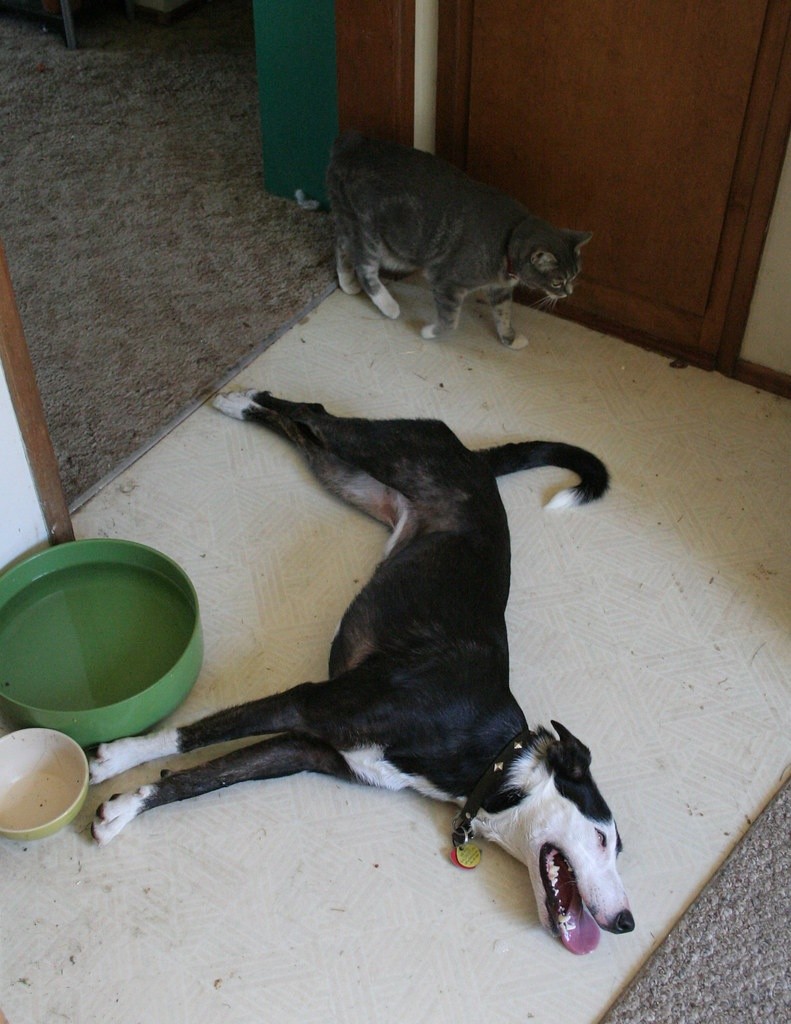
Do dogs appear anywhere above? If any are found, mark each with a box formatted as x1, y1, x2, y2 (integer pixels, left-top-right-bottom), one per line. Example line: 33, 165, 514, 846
86, 385, 637, 955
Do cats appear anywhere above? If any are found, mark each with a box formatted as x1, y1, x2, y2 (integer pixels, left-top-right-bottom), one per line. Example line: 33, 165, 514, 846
325, 129, 595, 351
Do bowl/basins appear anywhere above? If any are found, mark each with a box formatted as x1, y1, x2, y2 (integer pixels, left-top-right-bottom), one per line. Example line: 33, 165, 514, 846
0, 727, 90, 840
0, 538, 204, 747
